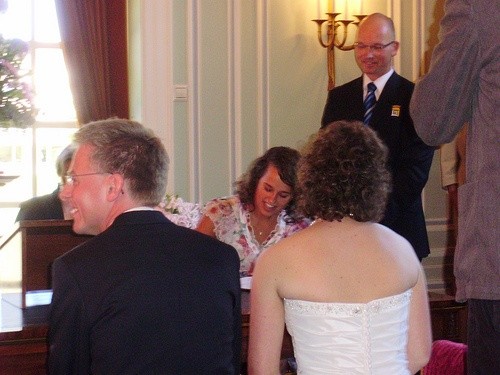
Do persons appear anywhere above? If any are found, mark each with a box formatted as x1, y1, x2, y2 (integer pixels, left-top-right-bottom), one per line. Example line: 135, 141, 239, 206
409, 0, 500, 375
45, 117, 242, 375
15, 144, 77, 221
247, 120, 432, 375
193, 146, 312, 279
320, 13, 435, 263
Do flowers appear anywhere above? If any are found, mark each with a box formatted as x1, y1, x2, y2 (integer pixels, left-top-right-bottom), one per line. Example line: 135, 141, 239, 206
0, 0, 41, 130
157, 194, 203, 229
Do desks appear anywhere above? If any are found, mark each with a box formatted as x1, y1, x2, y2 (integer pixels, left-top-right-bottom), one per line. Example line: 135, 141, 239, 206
0, 276, 472, 375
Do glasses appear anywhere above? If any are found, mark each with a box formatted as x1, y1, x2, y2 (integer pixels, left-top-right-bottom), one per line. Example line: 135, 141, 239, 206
352, 40, 394, 52
64, 172, 124, 194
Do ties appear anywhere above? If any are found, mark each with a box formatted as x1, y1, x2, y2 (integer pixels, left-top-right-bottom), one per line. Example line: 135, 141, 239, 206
363, 83, 377, 126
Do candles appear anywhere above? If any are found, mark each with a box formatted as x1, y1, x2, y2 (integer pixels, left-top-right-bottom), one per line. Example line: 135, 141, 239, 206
361, 0, 363, 14
316, 0, 320, 19
343, 0, 348, 19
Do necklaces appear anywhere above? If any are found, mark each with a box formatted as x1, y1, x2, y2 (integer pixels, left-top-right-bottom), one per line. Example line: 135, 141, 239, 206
254, 211, 275, 235
